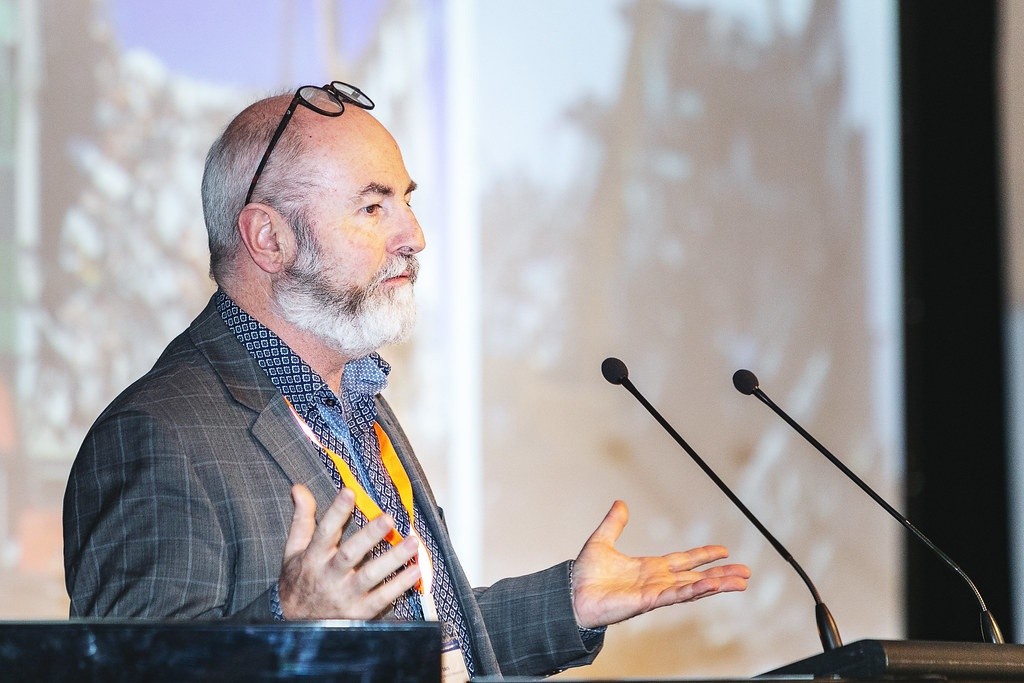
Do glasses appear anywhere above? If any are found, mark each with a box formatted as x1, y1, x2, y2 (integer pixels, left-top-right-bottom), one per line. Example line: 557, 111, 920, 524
245, 80, 375, 208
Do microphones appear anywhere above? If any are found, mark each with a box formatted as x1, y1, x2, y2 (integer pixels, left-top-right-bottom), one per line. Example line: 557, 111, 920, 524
733, 369, 1006, 645
600, 358, 842, 655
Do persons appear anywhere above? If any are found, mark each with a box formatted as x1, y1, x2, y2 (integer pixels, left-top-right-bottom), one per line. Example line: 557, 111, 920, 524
63, 92, 750, 682
